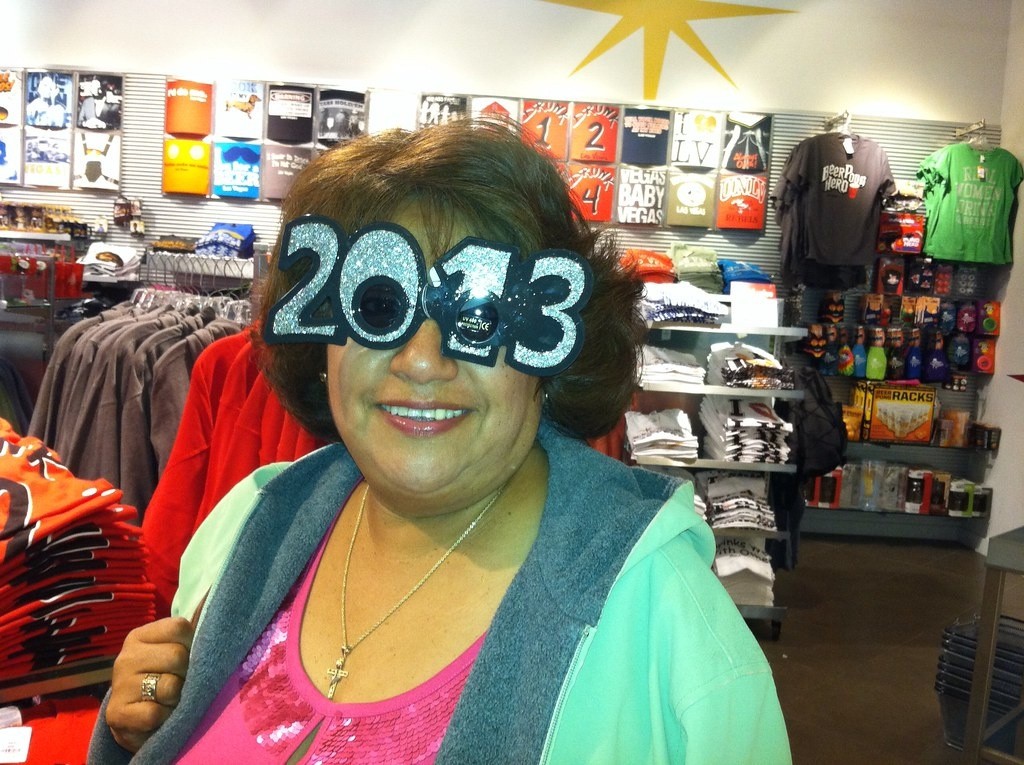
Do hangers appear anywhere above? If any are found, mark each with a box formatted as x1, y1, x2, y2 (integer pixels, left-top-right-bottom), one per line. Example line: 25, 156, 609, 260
131, 250, 254, 326
828, 110, 852, 134
959, 121, 992, 151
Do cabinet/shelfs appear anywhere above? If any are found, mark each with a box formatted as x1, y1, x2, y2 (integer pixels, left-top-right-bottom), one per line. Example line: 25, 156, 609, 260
637, 294, 808, 641
800, 441, 995, 542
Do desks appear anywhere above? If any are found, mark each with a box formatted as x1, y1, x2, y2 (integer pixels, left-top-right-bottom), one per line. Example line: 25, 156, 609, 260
963, 526, 1024, 765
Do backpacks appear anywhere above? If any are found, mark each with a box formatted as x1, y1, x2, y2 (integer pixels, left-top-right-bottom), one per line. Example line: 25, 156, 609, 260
784, 363, 848, 483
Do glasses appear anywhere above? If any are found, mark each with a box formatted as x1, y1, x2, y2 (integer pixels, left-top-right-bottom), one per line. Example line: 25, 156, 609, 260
261, 213, 595, 378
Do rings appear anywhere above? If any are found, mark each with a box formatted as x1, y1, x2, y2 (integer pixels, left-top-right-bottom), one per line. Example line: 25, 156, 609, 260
141, 673, 162, 701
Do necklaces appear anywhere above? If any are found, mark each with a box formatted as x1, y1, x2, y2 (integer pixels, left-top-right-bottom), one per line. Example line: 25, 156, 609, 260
326, 481, 506, 702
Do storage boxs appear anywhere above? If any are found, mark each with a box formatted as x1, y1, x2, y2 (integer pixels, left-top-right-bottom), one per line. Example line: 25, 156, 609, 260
934, 615, 1024, 752
848, 380, 936, 446
877, 211, 923, 254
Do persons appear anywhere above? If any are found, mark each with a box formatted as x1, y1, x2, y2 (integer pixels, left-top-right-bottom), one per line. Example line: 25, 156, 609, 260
86, 110, 795, 765
